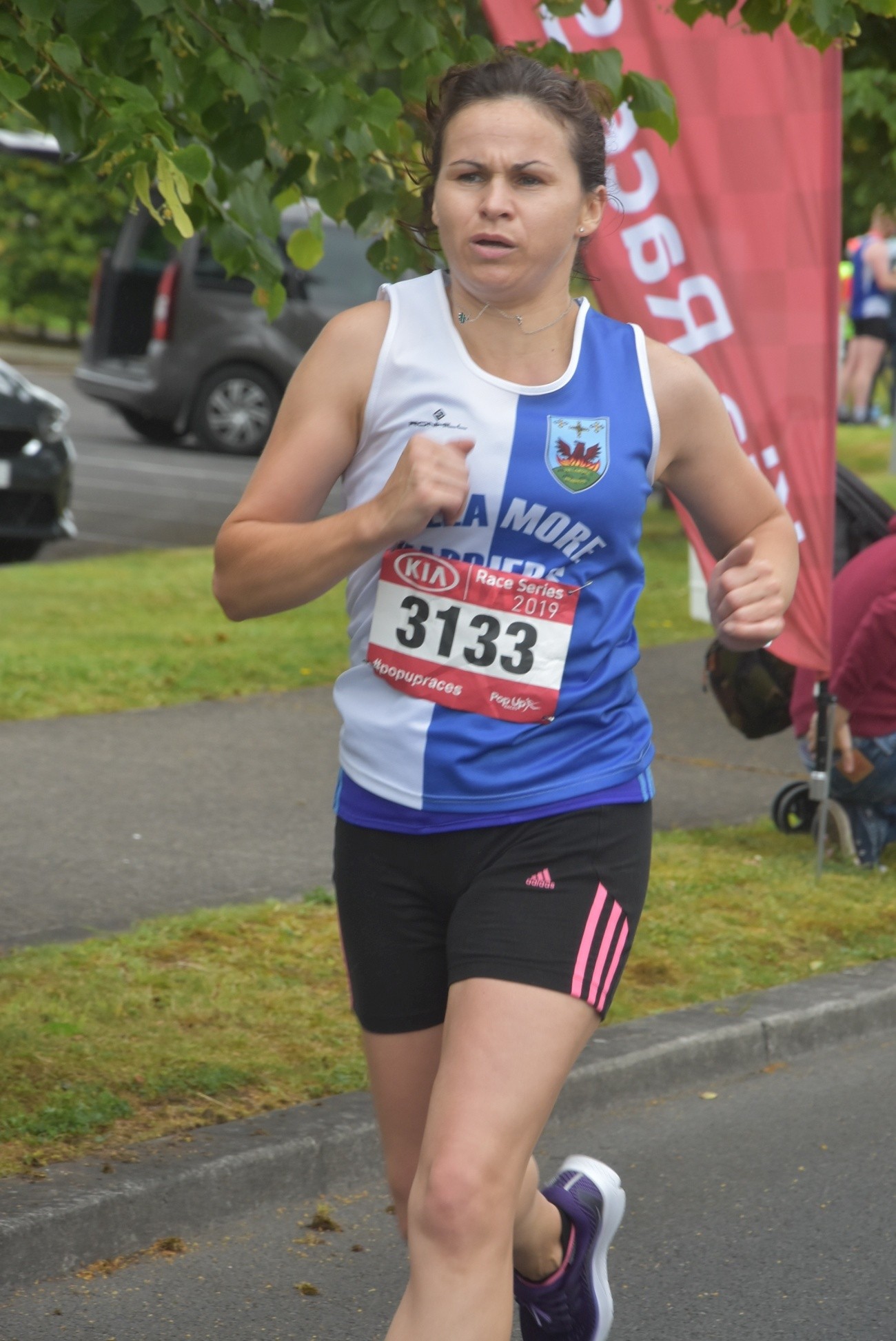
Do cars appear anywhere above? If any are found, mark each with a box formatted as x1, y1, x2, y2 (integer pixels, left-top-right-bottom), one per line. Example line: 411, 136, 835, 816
0, 357, 80, 563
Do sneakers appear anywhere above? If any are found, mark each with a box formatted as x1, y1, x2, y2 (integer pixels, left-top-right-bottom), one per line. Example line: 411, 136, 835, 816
812, 799, 890, 875
514, 1154, 626, 1341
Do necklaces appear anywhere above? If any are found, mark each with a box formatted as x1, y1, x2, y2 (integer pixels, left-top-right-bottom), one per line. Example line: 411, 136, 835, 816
456, 297, 578, 335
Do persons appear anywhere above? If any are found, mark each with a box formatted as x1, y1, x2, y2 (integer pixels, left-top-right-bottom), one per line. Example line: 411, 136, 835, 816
789, 513, 896, 870
211, 47, 801, 1341
837, 184, 896, 425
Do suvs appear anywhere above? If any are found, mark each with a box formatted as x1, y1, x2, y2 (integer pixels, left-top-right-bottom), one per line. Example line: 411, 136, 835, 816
71, 187, 452, 461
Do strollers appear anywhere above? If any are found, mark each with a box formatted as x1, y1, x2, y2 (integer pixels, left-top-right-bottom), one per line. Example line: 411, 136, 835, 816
703, 461, 896, 866
